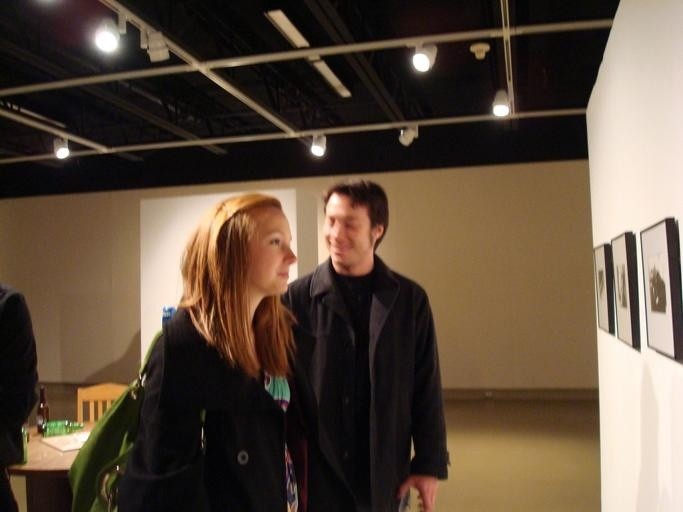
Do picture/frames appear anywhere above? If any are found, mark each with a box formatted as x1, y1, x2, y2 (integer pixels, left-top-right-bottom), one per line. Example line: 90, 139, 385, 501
610, 231, 639, 348
591, 243, 614, 333
640, 217, 683, 365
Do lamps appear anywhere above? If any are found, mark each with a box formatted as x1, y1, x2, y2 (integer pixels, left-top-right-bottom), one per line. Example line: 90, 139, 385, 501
412, 41, 438, 72
94, 10, 127, 53
310, 134, 327, 157
491, 90, 511, 118
398, 124, 419, 146
139, 25, 170, 63
53, 137, 70, 160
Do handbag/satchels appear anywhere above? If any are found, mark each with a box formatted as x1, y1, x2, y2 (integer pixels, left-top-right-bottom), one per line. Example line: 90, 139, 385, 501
68, 375, 145, 512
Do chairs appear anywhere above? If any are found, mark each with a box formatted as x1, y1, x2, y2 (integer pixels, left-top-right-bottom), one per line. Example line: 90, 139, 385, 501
77, 382, 129, 423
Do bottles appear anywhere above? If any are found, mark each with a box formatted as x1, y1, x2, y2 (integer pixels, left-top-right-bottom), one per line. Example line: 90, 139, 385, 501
36, 386, 84, 437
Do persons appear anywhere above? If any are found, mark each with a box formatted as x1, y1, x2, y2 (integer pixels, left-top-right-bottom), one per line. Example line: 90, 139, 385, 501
280, 181, 450, 512
0, 288, 36, 512
120, 192, 300, 512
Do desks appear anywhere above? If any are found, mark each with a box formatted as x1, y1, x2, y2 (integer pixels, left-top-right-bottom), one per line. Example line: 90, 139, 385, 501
7, 422, 102, 512
